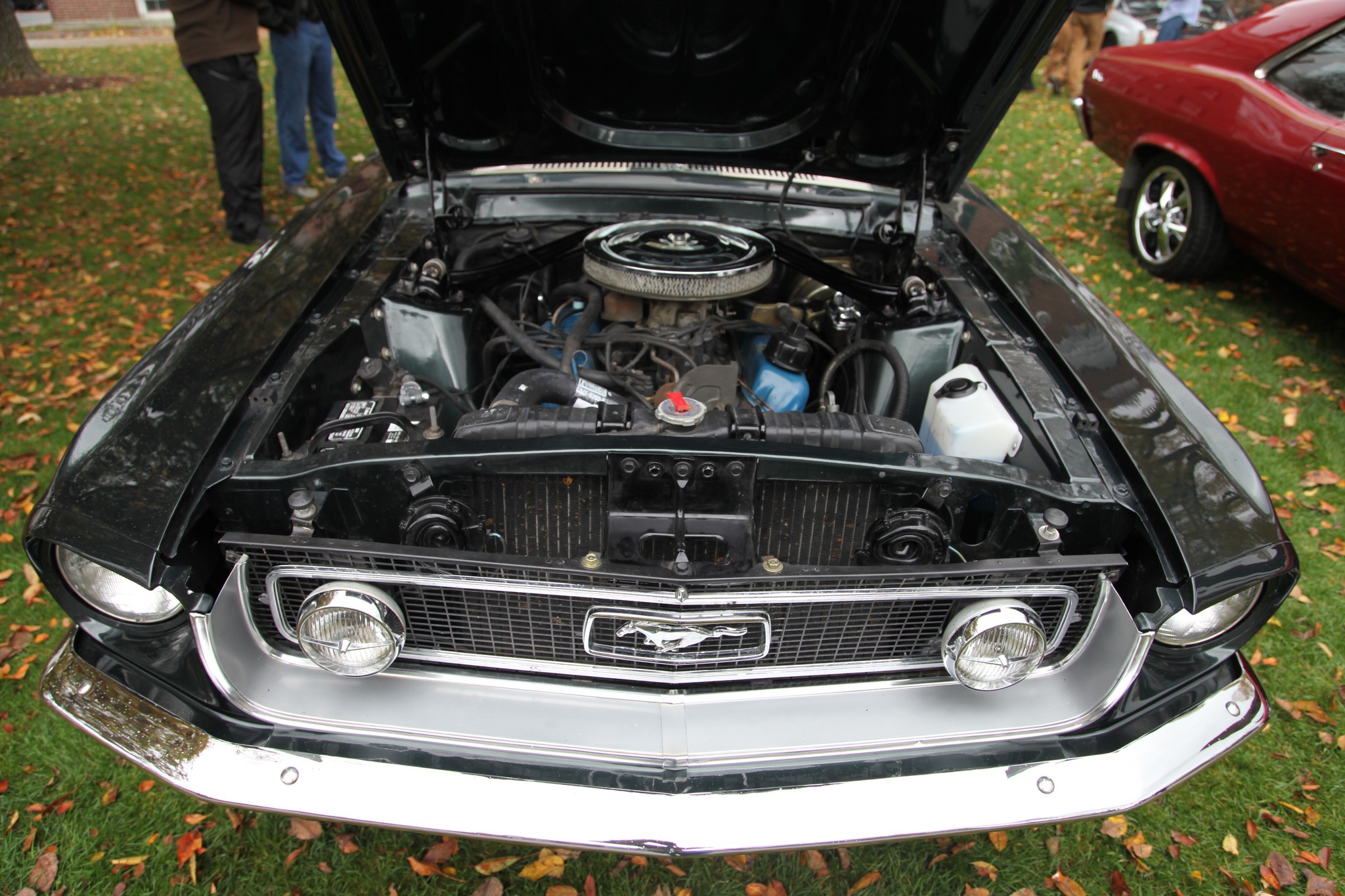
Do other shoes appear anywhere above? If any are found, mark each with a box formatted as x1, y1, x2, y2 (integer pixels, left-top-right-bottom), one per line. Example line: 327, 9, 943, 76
263, 218, 279, 230
230, 227, 275, 246
287, 185, 319, 199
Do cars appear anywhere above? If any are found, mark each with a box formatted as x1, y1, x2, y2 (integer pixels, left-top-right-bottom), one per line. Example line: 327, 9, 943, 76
21, 0, 1301, 849
1101, 0, 1290, 54
1072, 0, 1345, 317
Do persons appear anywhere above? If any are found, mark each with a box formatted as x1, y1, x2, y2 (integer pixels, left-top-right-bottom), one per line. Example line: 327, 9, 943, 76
255, 0, 348, 198
164, 0, 276, 244
1155, 0, 1203, 42
1019, 0, 1114, 106
